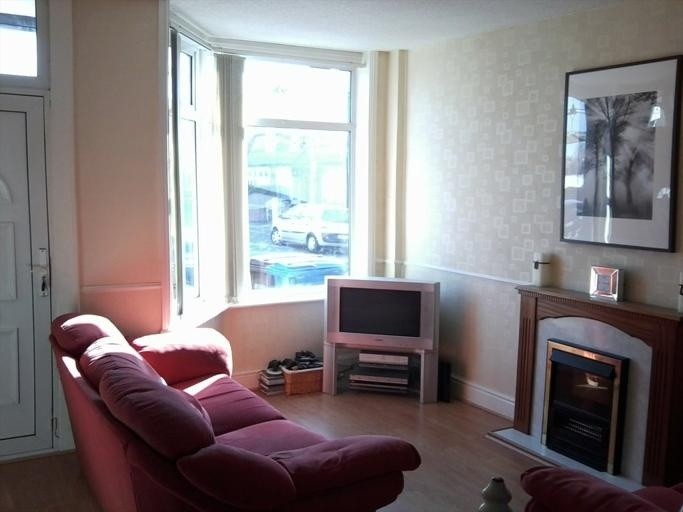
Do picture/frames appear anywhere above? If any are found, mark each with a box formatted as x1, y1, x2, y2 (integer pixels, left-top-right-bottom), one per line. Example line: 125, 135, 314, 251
560, 54, 682, 253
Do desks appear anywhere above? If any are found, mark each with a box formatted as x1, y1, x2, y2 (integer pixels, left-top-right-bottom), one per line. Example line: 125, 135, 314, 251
323, 339, 439, 405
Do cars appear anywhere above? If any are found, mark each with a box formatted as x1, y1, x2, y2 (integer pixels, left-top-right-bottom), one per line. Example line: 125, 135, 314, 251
269, 202, 349, 255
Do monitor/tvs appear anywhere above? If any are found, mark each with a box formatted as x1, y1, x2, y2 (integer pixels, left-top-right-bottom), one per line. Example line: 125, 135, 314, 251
325, 278, 439, 350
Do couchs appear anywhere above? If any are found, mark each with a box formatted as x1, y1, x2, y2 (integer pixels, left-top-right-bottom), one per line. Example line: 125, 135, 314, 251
47, 311, 421, 511
521, 464, 683, 512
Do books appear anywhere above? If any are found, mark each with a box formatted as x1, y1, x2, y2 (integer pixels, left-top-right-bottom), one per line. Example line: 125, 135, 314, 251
349, 351, 410, 391
258, 370, 285, 396
